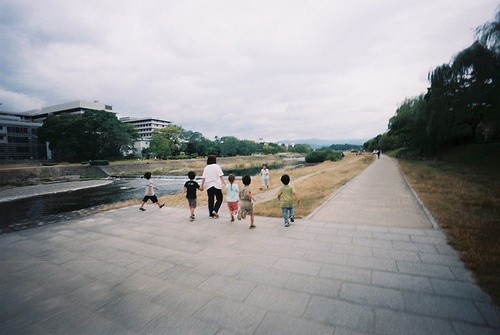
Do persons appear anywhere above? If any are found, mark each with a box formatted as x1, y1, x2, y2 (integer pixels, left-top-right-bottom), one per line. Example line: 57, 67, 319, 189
377, 149, 380, 159
224, 174, 239, 222
277, 174, 296, 227
139, 172, 166, 211
237, 175, 256, 229
201, 155, 226, 219
180, 171, 201, 220
261, 163, 269, 189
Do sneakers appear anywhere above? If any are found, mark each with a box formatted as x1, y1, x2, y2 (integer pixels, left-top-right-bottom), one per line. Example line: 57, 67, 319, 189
212, 211, 219, 218
290, 217, 294, 222
231, 215, 235, 222
209, 216, 214, 219
285, 222, 290, 227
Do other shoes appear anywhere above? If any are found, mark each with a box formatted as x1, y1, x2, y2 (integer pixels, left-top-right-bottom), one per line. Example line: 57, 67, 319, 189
160, 204, 165, 208
140, 208, 146, 211
190, 214, 195, 221
249, 225, 256, 229
237, 209, 242, 220
